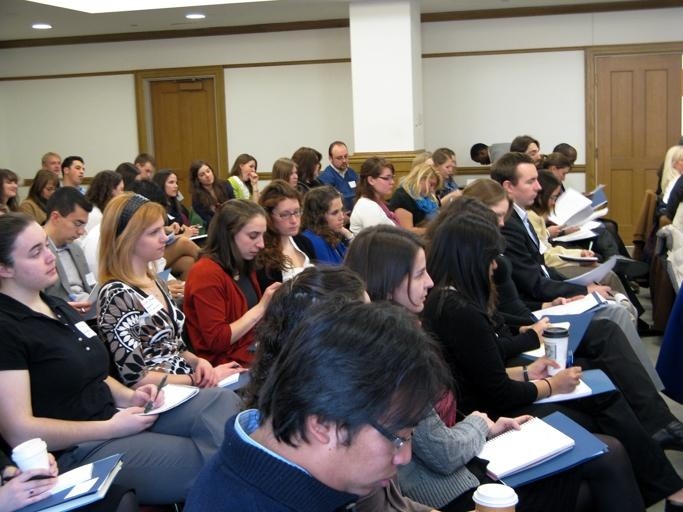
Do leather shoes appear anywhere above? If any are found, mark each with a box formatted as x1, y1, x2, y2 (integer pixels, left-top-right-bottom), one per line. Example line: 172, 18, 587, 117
650, 419, 683, 452
664, 498, 683, 512
607, 254, 650, 277
637, 317, 663, 338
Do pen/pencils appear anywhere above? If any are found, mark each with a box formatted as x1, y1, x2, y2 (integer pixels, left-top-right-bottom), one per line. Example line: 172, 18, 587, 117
566, 350, 573, 369
144, 375, 167, 413
69, 294, 85, 313
593, 280, 614, 297
192, 226, 203, 229
588, 240, 594, 251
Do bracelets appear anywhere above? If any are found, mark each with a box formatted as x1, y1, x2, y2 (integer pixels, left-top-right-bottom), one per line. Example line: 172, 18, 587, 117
523, 365, 529, 382
538, 377, 552, 399
187, 374, 194, 386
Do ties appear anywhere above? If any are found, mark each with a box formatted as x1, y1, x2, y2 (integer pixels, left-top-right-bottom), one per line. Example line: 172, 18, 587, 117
526, 218, 541, 251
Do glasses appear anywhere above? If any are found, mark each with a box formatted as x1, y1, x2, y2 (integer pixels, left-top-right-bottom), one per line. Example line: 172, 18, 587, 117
374, 173, 397, 181
44, 185, 57, 192
363, 417, 416, 451
271, 207, 305, 219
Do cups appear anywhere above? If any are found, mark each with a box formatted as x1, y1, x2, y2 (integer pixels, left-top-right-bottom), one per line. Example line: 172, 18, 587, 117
11, 438, 50, 472
471, 482, 519, 512
541, 326, 569, 376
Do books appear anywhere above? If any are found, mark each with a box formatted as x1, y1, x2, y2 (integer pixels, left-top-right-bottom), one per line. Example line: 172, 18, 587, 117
15, 452, 124, 511
35, 459, 123, 511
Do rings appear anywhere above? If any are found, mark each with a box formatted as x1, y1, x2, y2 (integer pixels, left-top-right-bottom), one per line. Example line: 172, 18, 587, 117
29, 489, 33, 495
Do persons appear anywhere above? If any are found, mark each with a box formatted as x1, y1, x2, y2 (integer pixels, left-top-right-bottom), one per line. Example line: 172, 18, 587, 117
93, 190, 250, 438
341, 222, 648, 511
241, 263, 443, 511
417, 207, 683, 512
184, 295, 449, 510
0, 447, 59, 511
0, 212, 238, 505
1, 133, 683, 355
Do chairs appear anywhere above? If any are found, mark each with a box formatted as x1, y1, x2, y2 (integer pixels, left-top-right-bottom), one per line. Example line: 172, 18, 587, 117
634, 186, 678, 353
